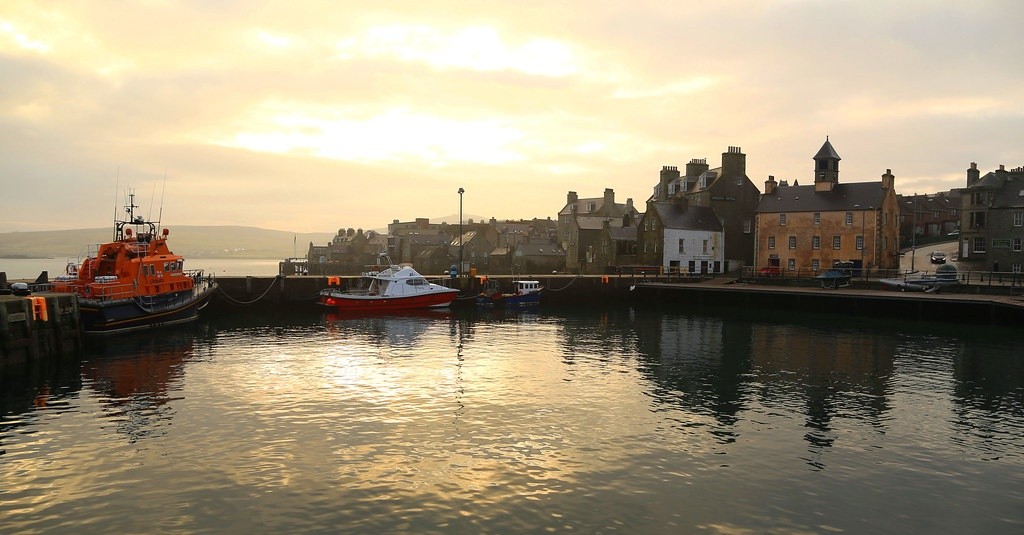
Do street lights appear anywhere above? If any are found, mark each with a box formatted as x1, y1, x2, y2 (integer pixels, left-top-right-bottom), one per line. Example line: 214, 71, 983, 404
907, 195, 921, 271
855, 204, 876, 281
458, 187, 466, 262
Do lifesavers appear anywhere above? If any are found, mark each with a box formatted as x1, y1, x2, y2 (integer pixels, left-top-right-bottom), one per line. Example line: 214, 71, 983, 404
79, 282, 96, 299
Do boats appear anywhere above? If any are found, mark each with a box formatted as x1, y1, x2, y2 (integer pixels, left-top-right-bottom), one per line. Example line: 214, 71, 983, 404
312, 262, 461, 310
473, 278, 547, 304
47, 188, 219, 341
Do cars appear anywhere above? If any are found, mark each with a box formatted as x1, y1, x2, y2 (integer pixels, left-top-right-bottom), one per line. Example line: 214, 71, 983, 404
758, 266, 780, 277
931, 251, 946, 263
948, 229, 962, 237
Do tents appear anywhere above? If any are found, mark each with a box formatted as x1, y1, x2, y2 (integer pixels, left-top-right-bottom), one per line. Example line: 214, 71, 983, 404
814, 271, 851, 289
